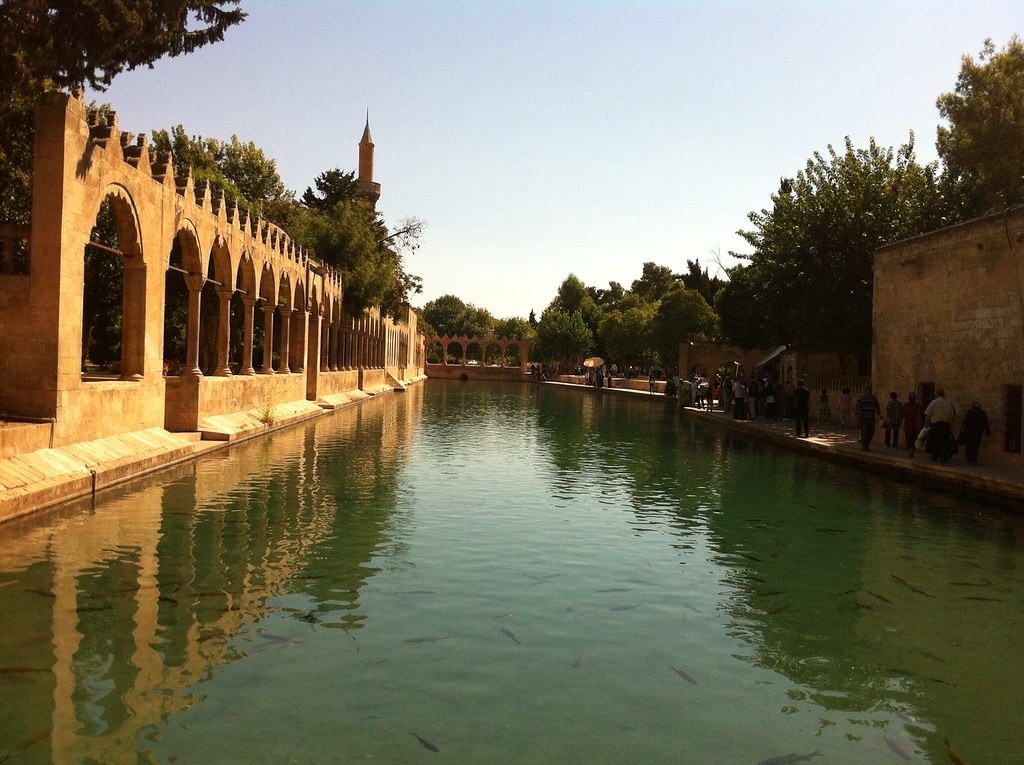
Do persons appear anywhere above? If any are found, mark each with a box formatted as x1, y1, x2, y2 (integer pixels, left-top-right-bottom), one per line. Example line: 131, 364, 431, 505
689, 361, 791, 421
854, 386, 881, 451
531, 354, 679, 395
924, 390, 956, 465
958, 398, 991, 467
794, 379, 810, 438
816, 389, 831, 425
882, 391, 929, 457
837, 388, 852, 430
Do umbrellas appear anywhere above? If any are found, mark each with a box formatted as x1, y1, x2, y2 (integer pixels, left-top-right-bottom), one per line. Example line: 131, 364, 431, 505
583, 357, 604, 371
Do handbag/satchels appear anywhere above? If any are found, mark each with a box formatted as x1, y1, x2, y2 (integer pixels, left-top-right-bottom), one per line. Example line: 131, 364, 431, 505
915, 427, 931, 454
957, 427, 967, 445
940, 431, 958, 464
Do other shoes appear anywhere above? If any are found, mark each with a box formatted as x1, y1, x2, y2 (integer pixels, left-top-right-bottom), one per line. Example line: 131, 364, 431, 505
805, 434, 809, 438
796, 433, 800, 437
